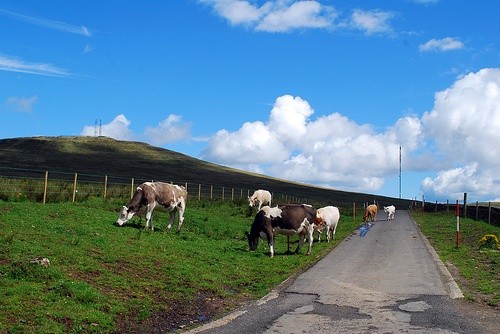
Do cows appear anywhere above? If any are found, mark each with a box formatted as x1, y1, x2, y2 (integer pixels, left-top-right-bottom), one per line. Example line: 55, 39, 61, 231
313, 206, 340, 243
363, 205, 377, 222
384, 205, 395, 221
244, 203, 317, 258
115, 181, 209, 234
248, 189, 272, 212
